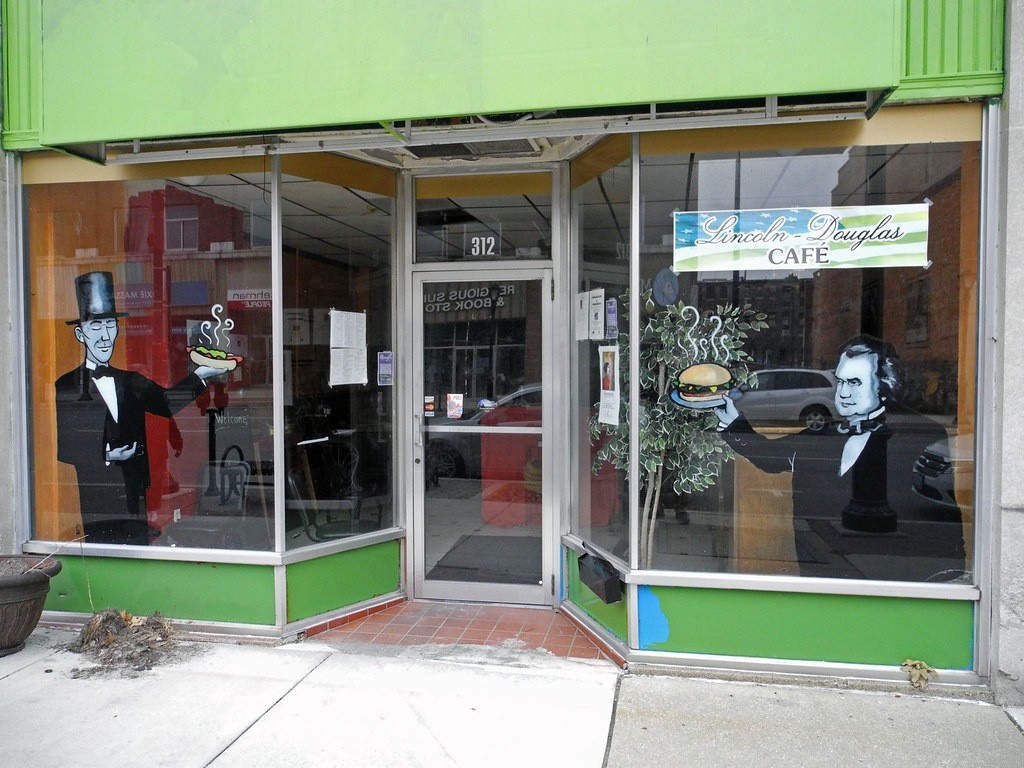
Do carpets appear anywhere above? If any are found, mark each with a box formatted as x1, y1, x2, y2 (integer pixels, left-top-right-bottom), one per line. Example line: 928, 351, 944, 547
425, 532, 543, 587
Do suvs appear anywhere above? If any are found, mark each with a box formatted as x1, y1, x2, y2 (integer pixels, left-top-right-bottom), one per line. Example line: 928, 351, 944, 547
731, 368, 844, 436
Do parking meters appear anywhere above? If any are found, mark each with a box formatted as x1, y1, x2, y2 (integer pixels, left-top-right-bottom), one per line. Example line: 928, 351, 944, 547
194, 374, 230, 498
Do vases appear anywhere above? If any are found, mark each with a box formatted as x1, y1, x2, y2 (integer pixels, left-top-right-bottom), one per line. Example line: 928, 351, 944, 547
0, 554, 63, 658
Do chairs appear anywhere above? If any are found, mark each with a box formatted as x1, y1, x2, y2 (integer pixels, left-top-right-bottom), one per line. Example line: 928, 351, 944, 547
168, 461, 251, 548
287, 469, 379, 542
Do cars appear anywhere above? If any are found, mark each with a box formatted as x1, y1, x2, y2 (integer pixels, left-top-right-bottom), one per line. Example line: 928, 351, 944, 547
428, 381, 542, 488
911, 432, 974, 511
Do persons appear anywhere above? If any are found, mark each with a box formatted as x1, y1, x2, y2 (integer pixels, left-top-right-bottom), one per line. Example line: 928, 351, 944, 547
646, 450, 690, 525
128, 363, 185, 524
